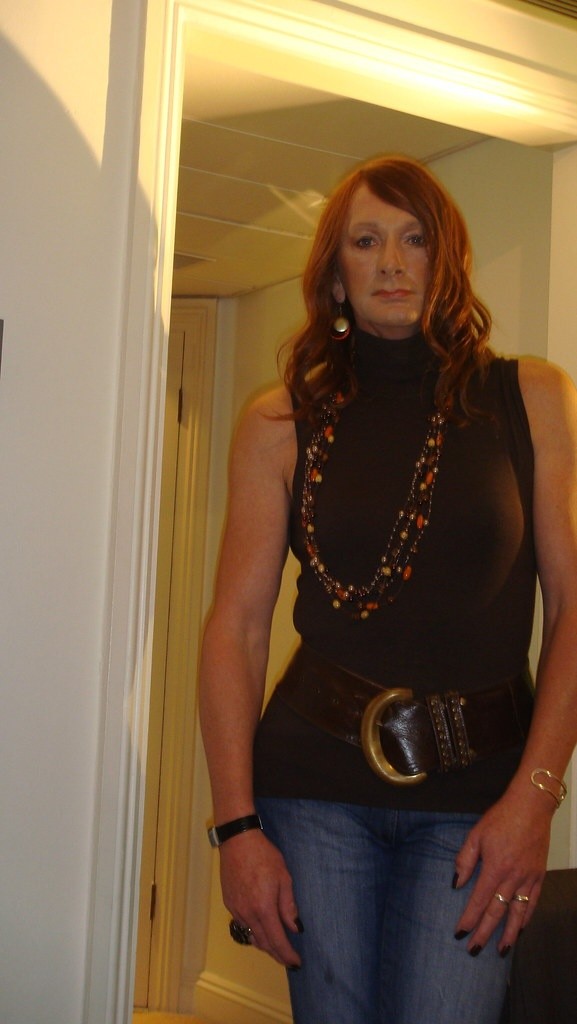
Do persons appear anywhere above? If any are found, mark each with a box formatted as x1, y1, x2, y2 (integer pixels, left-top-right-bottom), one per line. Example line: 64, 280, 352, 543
195, 159, 577, 1024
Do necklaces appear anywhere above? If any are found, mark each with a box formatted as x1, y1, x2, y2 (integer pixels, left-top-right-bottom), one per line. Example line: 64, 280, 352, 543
302, 347, 448, 623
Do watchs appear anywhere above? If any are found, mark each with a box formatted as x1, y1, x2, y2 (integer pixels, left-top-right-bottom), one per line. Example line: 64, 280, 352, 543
206, 813, 264, 848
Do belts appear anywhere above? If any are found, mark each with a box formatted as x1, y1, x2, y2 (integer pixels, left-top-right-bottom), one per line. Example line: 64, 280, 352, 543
273, 641, 536, 786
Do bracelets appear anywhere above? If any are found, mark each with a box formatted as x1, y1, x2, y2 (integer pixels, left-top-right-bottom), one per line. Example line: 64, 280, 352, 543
531, 767, 568, 810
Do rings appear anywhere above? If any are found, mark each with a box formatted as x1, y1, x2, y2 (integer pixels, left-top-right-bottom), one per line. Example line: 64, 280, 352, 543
229, 919, 252, 946
494, 892, 510, 905
513, 894, 529, 902
